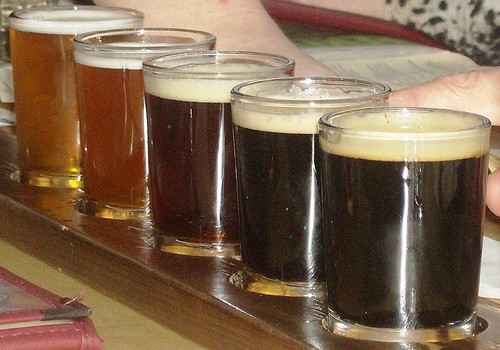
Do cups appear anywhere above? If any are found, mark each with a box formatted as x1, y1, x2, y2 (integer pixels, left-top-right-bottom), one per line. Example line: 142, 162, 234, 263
317, 106, 492, 342
74, 28, 217, 219
144, 50, 296, 258
230, 76, 391, 296
0, 0, 80, 125
11, 6, 145, 190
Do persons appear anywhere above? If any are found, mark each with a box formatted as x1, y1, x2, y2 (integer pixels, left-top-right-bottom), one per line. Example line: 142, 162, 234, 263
94, 0, 500, 218
